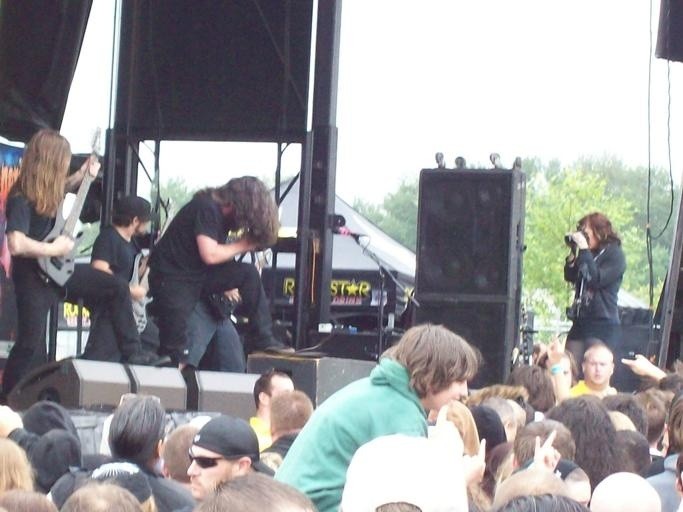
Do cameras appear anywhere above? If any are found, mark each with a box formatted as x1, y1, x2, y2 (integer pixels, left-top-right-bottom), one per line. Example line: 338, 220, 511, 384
565, 232, 589, 247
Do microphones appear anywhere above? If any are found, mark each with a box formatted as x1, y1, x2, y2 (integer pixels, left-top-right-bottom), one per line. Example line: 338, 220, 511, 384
332, 226, 353, 235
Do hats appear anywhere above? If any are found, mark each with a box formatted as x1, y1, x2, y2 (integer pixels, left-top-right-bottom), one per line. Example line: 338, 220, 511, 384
116, 197, 157, 220
192, 414, 260, 465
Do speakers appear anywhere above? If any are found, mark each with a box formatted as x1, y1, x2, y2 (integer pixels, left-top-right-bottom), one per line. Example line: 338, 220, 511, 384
124, 362, 187, 412
411, 298, 521, 390
245, 352, 375, 411
411, 166, 525, 298
188, 369, 262, 423
7, 356, 129, 412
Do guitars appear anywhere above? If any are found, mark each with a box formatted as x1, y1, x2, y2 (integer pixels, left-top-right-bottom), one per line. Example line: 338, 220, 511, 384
128, 197, 173, 334
37, 126, 103, 287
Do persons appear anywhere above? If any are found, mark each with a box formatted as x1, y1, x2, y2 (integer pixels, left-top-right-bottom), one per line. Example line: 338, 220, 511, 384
176, 299, 248, 373
562, 212, 627, 386
2, 129, 171, 404
148, 176, 296, 365
79, 197, 159, 361
1, 324, 683, 511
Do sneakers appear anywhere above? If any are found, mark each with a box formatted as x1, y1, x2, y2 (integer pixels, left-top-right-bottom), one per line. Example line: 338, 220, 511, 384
120, 351, 173, 366
252, 336, 296, 355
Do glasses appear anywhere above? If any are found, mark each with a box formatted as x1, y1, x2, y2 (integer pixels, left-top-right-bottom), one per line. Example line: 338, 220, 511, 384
117, 393, 161, 408
186, 447, 255, 468
